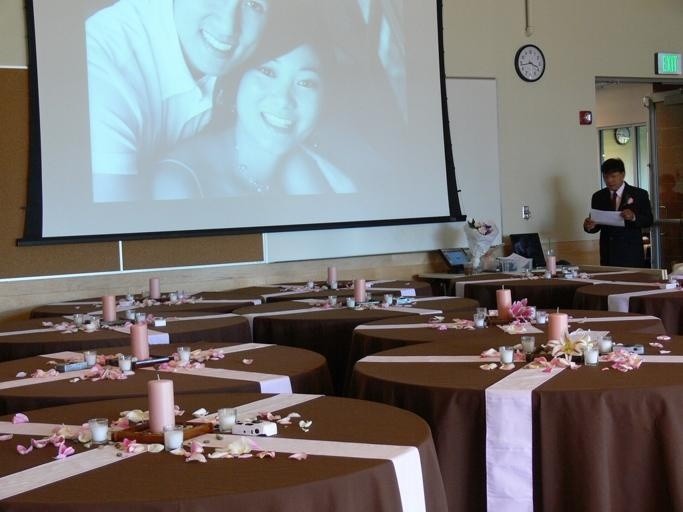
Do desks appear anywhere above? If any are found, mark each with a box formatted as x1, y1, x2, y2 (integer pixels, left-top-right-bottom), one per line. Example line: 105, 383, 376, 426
0, 264, 682, 512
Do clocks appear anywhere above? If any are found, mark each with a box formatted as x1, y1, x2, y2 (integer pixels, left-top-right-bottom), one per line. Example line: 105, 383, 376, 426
615, 127, 631, 145
514, 44, 546, 82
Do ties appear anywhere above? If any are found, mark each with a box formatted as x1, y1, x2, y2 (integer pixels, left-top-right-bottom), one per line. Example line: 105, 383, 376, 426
610, 191, 617, 215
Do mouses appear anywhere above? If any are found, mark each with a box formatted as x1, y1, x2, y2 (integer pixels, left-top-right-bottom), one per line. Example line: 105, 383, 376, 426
556, 260, 570, 265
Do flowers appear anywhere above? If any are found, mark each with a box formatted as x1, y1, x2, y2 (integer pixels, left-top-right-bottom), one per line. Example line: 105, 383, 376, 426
465, 219, 499, 269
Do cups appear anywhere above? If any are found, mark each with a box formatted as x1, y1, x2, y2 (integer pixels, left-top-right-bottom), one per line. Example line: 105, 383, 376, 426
463, 261, 473, 275
517, 264, 583, 281
305, 279, 394, 309
72, 286, 238, 454
471, 306, 613, 365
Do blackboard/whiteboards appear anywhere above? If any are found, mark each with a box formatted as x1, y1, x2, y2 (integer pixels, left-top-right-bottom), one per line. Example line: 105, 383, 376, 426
261, 76, 504, 264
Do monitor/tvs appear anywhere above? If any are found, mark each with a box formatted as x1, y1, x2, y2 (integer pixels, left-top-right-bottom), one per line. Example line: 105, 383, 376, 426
510, 232, 545, 268
440, 248, 468, 273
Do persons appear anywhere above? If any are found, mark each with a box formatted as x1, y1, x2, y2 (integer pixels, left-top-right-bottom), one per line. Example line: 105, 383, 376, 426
84, 0, 356, 202
583, 157, 654, 268
